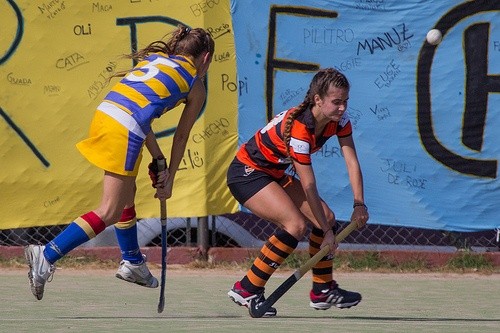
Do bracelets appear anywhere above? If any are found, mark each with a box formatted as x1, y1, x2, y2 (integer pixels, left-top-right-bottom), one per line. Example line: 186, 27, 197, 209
353, 203, 368, 211
324, 229, 333, 237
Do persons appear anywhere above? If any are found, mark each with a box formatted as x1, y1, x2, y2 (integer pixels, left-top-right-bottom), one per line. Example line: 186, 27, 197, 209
24, 24, 216, 300
226, 67, 370, 316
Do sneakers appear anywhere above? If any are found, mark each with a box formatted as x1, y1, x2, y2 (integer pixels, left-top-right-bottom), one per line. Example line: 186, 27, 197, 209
310, 280, 363, 310
24, 243, 56, 300
227, 280, 277, 316
116, 254, 159, 288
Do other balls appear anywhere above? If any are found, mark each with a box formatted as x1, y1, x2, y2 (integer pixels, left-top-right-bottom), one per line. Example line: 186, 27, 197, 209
426, 28, 443, 45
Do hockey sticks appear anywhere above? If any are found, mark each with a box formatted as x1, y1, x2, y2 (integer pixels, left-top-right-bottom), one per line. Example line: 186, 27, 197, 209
248, 218, 359, 320
153, 156, 167, 315
